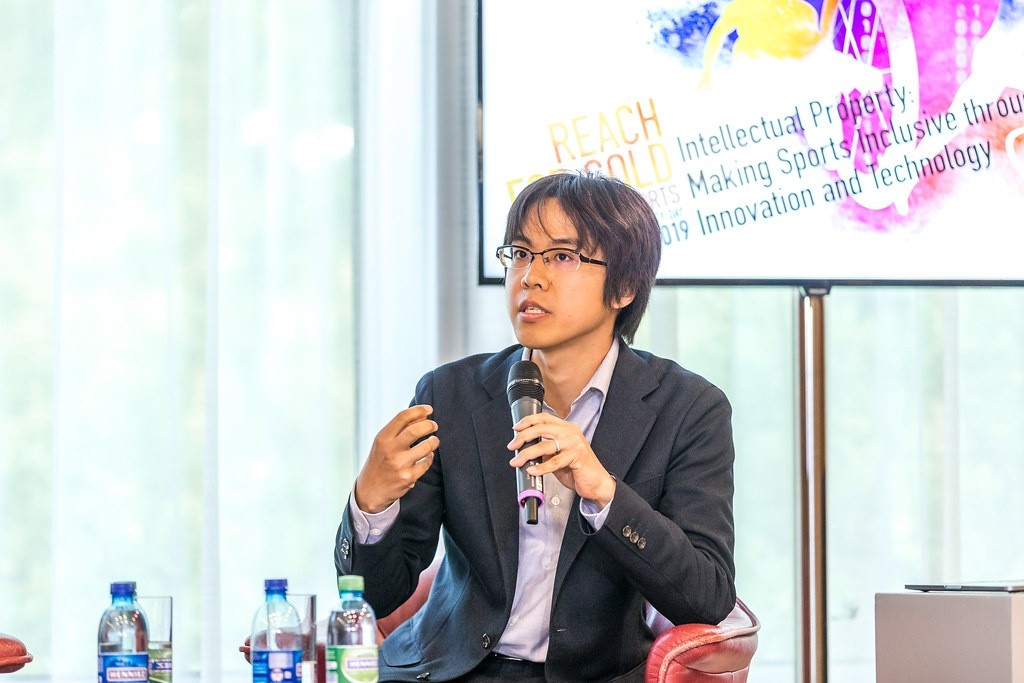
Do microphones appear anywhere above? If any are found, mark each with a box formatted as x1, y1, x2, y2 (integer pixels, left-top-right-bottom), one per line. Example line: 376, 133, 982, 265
506, 360, 545, 524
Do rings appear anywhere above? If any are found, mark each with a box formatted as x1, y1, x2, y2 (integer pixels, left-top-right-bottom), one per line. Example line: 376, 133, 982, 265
553, 439, 561, 453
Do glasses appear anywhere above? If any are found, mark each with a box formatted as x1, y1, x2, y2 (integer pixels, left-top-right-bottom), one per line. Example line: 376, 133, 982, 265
496, 243, 608, 275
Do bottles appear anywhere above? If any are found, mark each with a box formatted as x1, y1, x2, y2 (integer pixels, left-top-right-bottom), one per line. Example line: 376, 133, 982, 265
249, 578, 302, 682
324, 576, 380, 683
96, 581, 150, 683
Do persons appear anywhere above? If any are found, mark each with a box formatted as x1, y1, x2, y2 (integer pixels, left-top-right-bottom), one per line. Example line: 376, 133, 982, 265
333, 166, 737, 683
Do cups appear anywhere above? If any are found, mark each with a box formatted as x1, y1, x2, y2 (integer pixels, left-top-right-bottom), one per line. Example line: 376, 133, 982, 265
122, 597, 173, 683
266, 592, 317, 683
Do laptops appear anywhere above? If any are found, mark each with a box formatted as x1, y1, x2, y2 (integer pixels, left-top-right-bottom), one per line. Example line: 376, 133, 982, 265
905, 579, 1023, 592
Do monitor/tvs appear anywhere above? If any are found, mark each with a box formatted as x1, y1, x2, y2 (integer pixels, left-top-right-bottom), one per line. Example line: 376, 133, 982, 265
473, 0, 1024, 287
